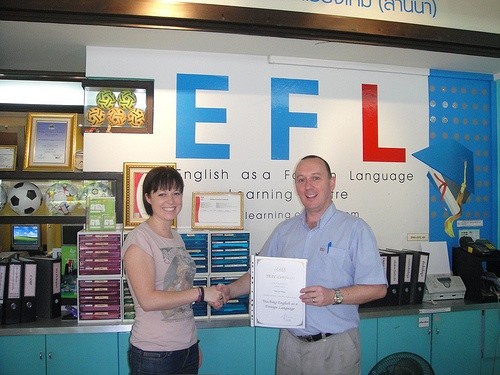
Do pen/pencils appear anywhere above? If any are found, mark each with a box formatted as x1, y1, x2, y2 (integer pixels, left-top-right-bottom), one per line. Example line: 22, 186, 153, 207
327, 242, 331, 253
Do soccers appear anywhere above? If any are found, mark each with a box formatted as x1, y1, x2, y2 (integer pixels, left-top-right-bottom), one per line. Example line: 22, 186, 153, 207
1, 180, 114, 215
86, 91, 146, 128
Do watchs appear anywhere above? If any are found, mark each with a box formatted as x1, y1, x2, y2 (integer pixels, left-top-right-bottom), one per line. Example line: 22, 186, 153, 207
333, 289, 343, 305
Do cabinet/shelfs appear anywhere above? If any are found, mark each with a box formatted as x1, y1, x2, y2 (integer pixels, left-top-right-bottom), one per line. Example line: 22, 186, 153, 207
78, 228, 252, 324
0, 307, 500, 375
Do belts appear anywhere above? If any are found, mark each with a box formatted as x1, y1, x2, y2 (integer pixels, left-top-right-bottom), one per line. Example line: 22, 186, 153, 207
297, 333, 331, 343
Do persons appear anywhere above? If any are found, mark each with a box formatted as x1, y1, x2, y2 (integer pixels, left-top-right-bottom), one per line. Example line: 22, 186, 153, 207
214, 155, 387, 375
120, 167, 228, 375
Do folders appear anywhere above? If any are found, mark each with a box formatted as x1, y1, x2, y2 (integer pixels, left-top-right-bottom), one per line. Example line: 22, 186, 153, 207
29, 255, 62, 319
0, 256, 38, 325
369, 248, 430, 307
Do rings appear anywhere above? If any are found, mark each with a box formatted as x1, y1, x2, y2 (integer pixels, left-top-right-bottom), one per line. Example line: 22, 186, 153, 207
313, 299, 315, 303
220, 296, 223, 300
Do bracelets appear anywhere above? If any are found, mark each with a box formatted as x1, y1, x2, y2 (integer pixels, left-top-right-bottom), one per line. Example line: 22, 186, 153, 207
196, 287, 204, 303
